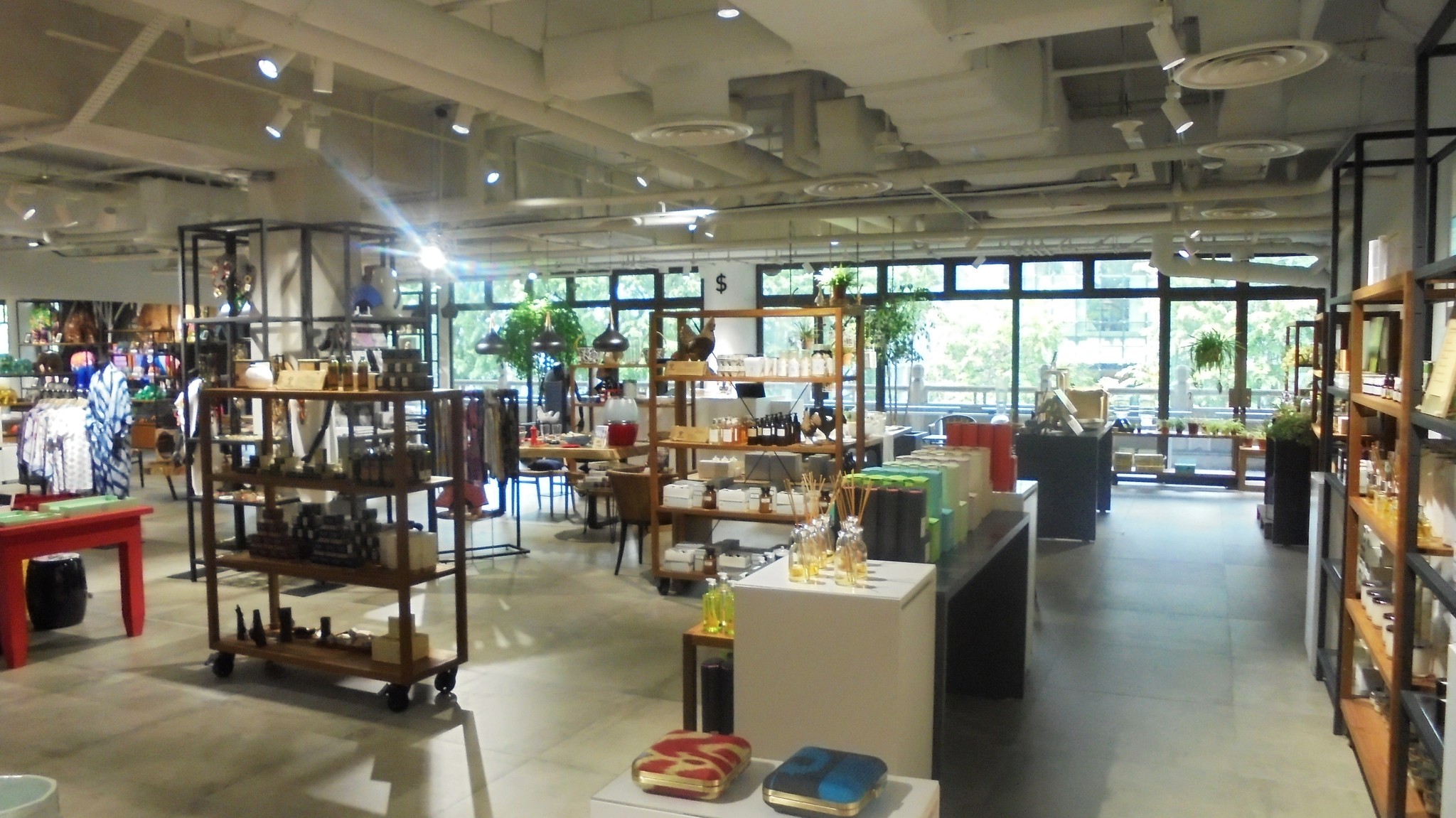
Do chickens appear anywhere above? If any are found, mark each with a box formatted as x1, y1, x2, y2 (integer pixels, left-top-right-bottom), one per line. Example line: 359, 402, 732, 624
800, 407, 821, 443
680, 317, 715, 361
537, 406, 560, 423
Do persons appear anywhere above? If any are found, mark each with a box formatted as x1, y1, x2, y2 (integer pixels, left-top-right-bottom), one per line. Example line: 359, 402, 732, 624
85, 361, 137, 499
538, 362, 585, 431
176, 378, 226, 498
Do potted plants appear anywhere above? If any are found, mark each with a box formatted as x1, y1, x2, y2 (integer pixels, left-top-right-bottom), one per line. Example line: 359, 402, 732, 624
1121, 331, 1310, 439
813, 269, 932, 368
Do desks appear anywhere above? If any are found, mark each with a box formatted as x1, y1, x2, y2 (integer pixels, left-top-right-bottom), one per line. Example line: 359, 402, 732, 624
519, 436, 650, 530
1112, 431, 1246, 490
1237, 445, 1268, 492
0, 495, 153, 670
1257, 436, 1321, 544
588, 421, 1113, 818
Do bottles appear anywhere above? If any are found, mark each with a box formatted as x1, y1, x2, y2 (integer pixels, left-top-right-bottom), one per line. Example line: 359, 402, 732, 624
758, 486, 773, 514
1365, 473, 1430, 544
704, 547, 716, 576
327, 355, 339, 391
348, 434, 432, 487
357, 356, 368, 392
342, 355, 353, 392
709, 416, 749, 445
788, 514, 868, 586
747, 412, 801, 446
1382, 373, 1395, 400
702, 484, 717, 510
245, 364, 274, 390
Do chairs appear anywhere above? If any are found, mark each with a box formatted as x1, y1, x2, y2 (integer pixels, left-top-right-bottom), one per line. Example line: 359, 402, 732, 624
511, 468, 575, 516
605, 469, 696, 574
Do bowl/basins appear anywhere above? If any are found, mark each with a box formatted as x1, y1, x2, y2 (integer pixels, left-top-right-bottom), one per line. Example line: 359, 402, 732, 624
846, 421, 873, 439
562, 434, 592, 446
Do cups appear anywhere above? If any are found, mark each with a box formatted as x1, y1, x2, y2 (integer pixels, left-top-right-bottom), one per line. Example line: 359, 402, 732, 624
540, 424, 550, 439
1360, 580, 1434, 678
0, 774, 60, 818
552, 424, 562, 437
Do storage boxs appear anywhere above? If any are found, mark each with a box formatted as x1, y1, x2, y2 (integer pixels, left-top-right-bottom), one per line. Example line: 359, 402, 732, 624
843, 410, 887, 437
129, 422, 180, 448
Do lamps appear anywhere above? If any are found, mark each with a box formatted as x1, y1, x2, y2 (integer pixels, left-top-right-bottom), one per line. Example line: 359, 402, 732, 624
636, 164, 718, 239
467, 233, 630, 353
1110, 0, 1201, 266
256, 47, 334, 151
451, 105, 501, 185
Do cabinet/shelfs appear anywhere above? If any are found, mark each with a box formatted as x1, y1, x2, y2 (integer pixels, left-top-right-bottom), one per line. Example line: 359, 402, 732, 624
200, 381, 468, 708
1284, 0, 1456, 818
176, 215, 433, 583
647, 308, 864, 581
14, 299, 177, 428
